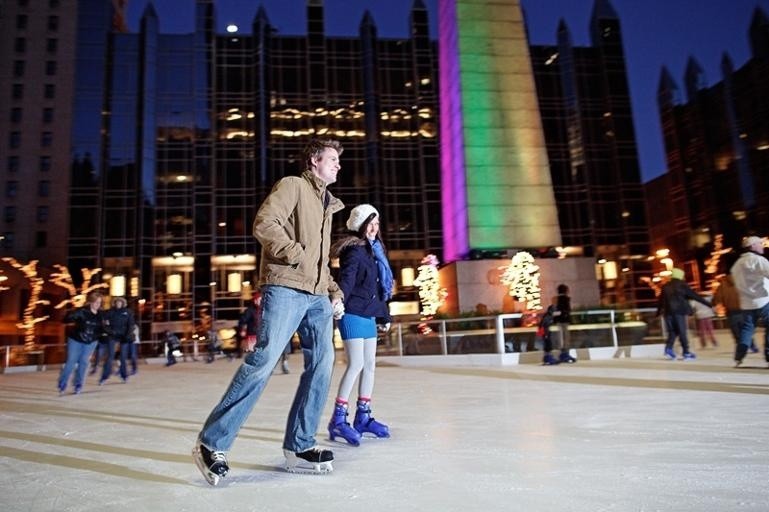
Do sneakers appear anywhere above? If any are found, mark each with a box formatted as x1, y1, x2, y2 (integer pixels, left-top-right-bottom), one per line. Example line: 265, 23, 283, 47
327, 404, 361, 439
200, 445, 229, 477
353, 402, 388, 433
57, 381, 82, 392
665, 348, 696, 358
543, 352, 575, 364
296, 446, 333, 463
90, 366, 137, 384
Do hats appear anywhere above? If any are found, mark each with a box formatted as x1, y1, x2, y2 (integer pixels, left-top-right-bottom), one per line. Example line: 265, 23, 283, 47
346, 203, 381, 232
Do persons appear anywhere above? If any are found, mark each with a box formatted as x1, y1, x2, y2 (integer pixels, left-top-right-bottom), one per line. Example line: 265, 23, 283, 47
731, 237, 769, 362
556, 284, 576, 363
537, 305, 559, 364
709, 258, 759, 353
197, 140, 345, 478
688, 288, 718, 347
655, 268, 714, 358
57, 291, 291, 394
329, 204, 395, 439
